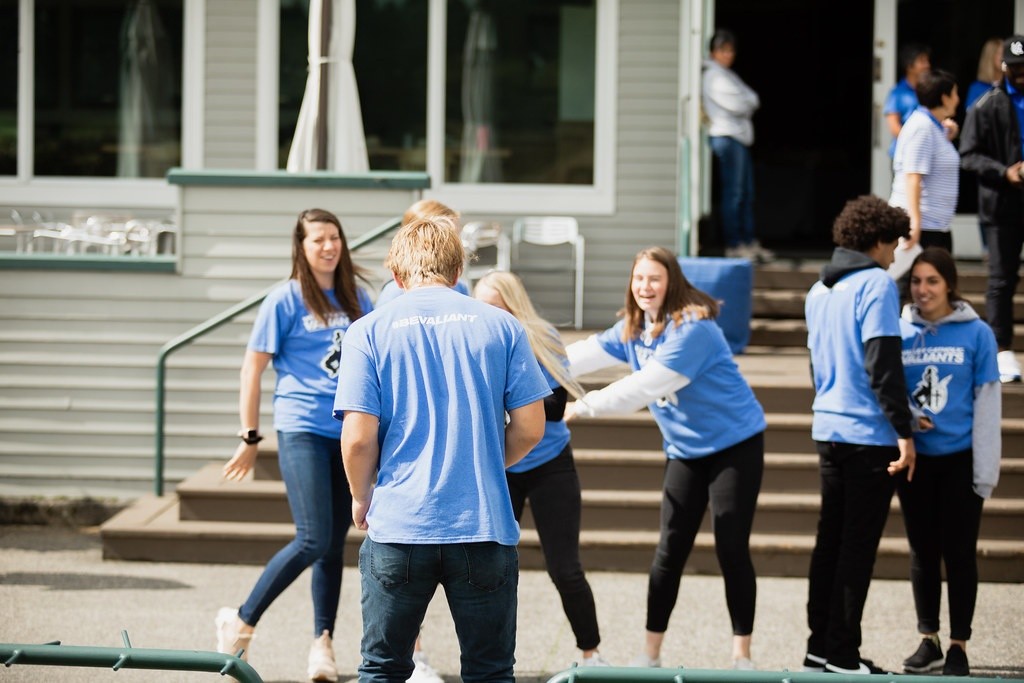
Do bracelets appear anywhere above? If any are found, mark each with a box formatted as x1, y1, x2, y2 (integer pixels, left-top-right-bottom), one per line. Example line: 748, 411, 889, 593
240, 436, 262, 445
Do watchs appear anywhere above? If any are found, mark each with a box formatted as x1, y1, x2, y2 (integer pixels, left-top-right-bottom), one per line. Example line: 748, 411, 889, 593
237, 428, 256, 439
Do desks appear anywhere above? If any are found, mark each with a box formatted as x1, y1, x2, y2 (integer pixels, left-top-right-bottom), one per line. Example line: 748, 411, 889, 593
104, 141, 181, 179
368, 144, 512, 180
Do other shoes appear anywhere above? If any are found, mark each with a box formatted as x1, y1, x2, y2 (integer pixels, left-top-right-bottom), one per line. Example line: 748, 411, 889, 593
724, 244, 772, 262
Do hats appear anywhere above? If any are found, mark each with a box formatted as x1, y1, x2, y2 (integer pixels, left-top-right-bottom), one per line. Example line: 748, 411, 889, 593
1001, 37, 1024, 65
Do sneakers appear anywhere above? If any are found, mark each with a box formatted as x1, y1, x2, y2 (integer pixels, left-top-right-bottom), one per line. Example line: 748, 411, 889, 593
405, 650, 445, 683
997, 350, 1022, 383
628, 653, 661, 668
308, 629, 338, 683
731, 657, 757, 670
802, 651, 875, 671
579, 649, 612, 667
822, 659, 900, 675
943, 643, 969, 676
215, 607, 254, 664
901, 635, 945, 673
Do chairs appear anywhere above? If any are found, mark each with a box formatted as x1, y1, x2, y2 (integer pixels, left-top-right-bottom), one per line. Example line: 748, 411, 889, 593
455, 213, 584, 331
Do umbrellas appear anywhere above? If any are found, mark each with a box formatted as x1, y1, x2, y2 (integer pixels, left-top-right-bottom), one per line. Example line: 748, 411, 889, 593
285, 0, 370, 176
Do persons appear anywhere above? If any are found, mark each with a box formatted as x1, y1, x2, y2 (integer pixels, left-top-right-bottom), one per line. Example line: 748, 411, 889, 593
332, 221, 554, 683
215, 209, 767, 683
702, 31, 773, 263
801, 193, 1002, 676
881, 35, 1024, 384
